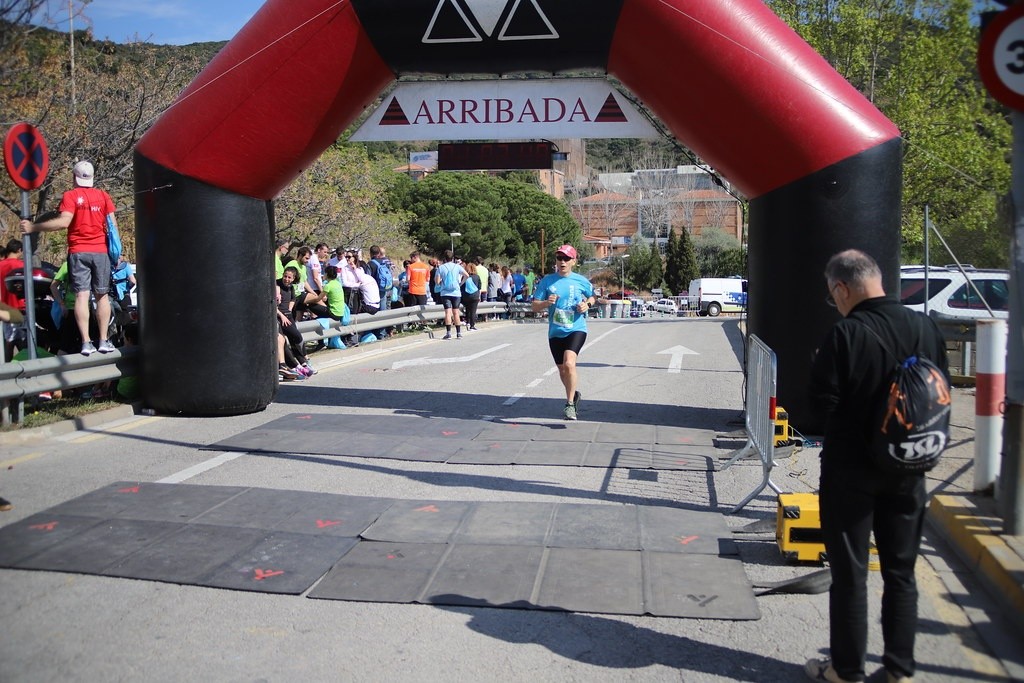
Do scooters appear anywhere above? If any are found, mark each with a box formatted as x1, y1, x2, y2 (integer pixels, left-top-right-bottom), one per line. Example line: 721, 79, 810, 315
3, 268, 139, 350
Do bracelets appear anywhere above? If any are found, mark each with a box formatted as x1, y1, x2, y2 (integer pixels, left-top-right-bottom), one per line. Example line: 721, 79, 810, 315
588, 303, 591, 308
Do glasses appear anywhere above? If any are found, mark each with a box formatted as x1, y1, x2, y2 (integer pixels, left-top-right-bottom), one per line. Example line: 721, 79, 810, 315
556, 256, 573, 261
826, 281, 849, 308
346, 256, 354, 259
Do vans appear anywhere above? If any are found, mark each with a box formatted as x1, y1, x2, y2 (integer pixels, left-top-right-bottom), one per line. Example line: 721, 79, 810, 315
688, 278, 747, 317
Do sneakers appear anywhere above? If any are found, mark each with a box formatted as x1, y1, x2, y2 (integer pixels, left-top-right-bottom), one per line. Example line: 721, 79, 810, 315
278, 339, 359, 381
567, 390, 581, 411
805, 657, 862, 683
866, 667, 912, 683
82, 340, 96, 353
98, 340, 115, 352
564, 404, 577, 420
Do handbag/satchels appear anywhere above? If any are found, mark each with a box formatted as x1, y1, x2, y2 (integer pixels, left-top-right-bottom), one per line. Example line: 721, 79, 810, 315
100, 188, 121, 265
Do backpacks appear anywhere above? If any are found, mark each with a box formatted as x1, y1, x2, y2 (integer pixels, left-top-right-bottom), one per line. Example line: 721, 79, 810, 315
465, 276, 478, 294
441, 263, 459, 292
847, 312, 950, 470
370, 259, 393, 291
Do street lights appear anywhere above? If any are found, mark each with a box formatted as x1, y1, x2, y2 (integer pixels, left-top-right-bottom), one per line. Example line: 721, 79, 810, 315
449, 233, 462, 262
621, 254, 630, 300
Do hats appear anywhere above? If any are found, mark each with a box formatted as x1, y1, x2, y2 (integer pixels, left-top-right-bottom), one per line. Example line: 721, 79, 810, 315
73, 161, 94, 188
555, 245, 576, 259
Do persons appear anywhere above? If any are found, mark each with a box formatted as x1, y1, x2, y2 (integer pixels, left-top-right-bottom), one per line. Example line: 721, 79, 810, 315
462, 263, 481, 331
532, 245, 596, 420
0, 239, 136, 360
805, 248, 950, 683
435, 250, 469, 339
20, 161, 121, 355
275, 239, 441, 383
470, 256, 546, 322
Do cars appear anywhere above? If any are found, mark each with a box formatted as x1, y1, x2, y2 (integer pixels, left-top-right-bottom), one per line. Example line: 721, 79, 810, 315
900, 263, 1010, 318
608, 291, 636, 300
645, 298, 679, 315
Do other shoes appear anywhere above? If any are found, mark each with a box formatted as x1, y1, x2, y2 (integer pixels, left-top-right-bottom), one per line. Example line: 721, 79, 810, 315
38, 392, 52, 401
81, 386, 113, 398
389, 317, 500, 339
